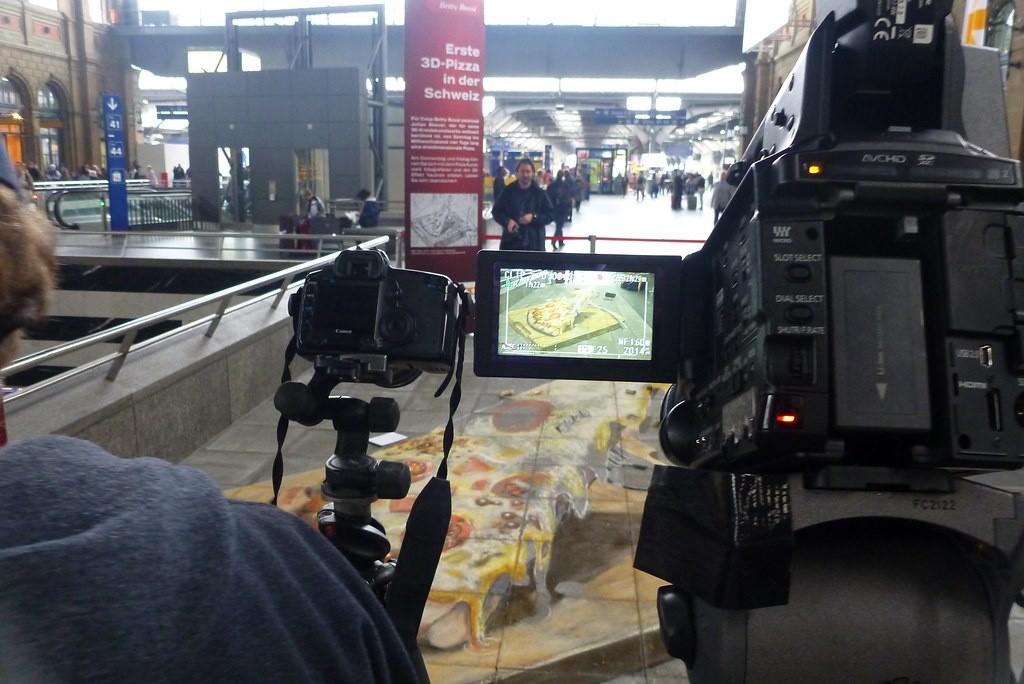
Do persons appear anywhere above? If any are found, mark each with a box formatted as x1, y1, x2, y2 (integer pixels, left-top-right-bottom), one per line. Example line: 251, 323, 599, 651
546, 170, 573, 250
14, 161, 107, 189
493, 166, 506, 200
0, 132, 417, 684
711, 172, 734, 224
146, 165, 159, 187
173, 164, 191, 179
356, 189, 380, 227
630, 169, 705, 210
564, 170, 584, 212
299, 186, 324, 218
127, 161, 141, 178
706, 173, 714, 190
535, 168, 551, 187
493, 158, 554, 250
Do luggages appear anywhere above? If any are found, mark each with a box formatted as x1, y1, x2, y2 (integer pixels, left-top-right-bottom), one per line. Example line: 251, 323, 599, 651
688, 196, 697, 210
672, 195, 680, 209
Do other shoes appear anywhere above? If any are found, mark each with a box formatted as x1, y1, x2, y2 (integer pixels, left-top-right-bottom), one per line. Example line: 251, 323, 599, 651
559, 242, 565, 247
552, 241, 557, 249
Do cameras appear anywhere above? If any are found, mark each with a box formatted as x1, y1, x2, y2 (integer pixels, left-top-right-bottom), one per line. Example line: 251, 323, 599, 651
288, 250, 463, 386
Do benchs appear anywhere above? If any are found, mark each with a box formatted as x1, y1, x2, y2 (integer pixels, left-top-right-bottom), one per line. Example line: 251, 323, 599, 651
343, 225, 405, 253
